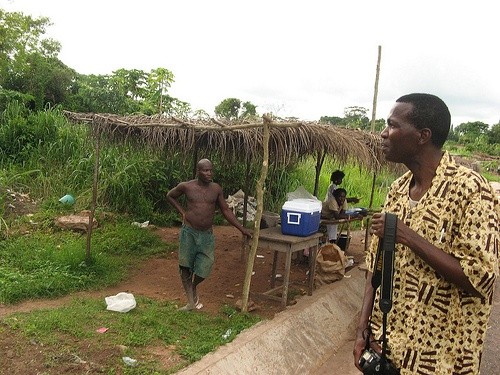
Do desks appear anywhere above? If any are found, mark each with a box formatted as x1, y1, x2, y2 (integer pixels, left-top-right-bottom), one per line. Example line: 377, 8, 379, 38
320, 218, 371, 254
247, 227, 324, 306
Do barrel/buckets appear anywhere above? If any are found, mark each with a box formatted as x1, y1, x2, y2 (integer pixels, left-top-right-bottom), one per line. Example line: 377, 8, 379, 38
337, 233, 350, 251
262, 210, 280, 228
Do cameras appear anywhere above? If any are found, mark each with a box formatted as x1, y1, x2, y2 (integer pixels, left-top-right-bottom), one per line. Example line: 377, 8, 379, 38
358, 348, 400, 375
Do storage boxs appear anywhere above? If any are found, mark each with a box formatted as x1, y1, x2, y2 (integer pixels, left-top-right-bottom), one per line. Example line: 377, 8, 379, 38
281, 198, 322, 237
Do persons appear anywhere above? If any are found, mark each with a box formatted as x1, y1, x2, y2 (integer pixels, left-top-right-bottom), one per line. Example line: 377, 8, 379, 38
351, 92, 499, 375
318, 188, 364, 222
166, 158, 255, 312
321, 170, 360, 243
